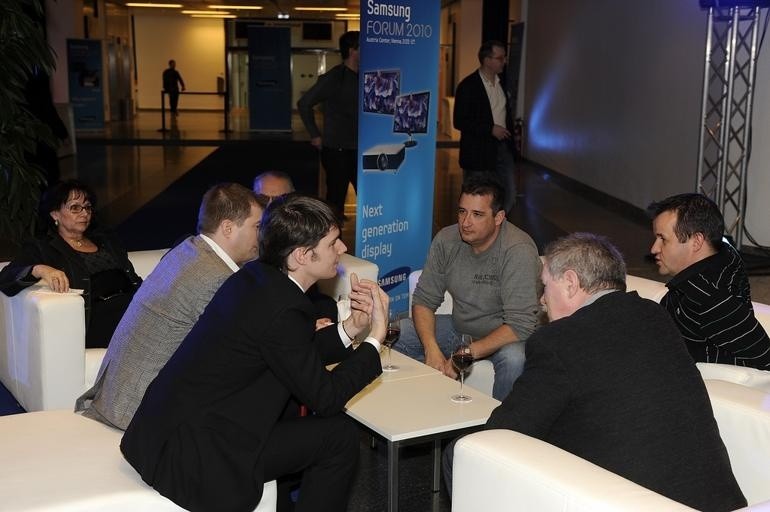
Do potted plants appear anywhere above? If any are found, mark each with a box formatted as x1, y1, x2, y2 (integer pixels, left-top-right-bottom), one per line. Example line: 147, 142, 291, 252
2, 0, 66, 262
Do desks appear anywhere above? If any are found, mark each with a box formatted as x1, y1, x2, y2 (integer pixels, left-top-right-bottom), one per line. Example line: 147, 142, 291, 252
326, 341, 504, 512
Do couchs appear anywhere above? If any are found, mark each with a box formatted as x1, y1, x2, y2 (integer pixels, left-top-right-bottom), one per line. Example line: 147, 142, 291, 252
448, 376, 767, 512
0, 245, 379, 413
0, 408, 279, 509
409, 255, 770, 338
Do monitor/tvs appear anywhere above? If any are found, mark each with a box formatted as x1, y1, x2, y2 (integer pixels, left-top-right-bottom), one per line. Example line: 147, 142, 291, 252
393, 92, 432, 148
361, 69, 401, 116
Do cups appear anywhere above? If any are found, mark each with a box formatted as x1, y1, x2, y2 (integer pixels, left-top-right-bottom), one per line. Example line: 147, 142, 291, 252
381, 309, 401, 372
450, 334, 473, 404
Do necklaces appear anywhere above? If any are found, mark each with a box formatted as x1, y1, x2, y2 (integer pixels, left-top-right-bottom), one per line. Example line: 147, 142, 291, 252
67, 239, 90, 247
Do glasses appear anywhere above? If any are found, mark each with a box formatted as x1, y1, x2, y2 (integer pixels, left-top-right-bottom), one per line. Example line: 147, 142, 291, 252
488, 55, 505, 61
67, 203, 94, 213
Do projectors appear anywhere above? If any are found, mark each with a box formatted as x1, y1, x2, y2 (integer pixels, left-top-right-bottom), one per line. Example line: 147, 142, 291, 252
361, 144, 407, 174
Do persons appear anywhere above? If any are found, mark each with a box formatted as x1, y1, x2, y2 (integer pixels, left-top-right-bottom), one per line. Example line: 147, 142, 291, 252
161, 57, 188, 122
118, 192, 391, 507
648, 195, 770, 371
452, 37, 527, 218
439, 229, 750, 512
4, 175, 146, 352
251, 168, 297, 211
72, 182, 264, 432
295, 29, 362, 242
388, 180, 548, 457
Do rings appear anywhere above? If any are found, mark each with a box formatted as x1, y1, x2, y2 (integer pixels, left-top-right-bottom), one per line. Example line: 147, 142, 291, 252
52, 277, 59, 280
